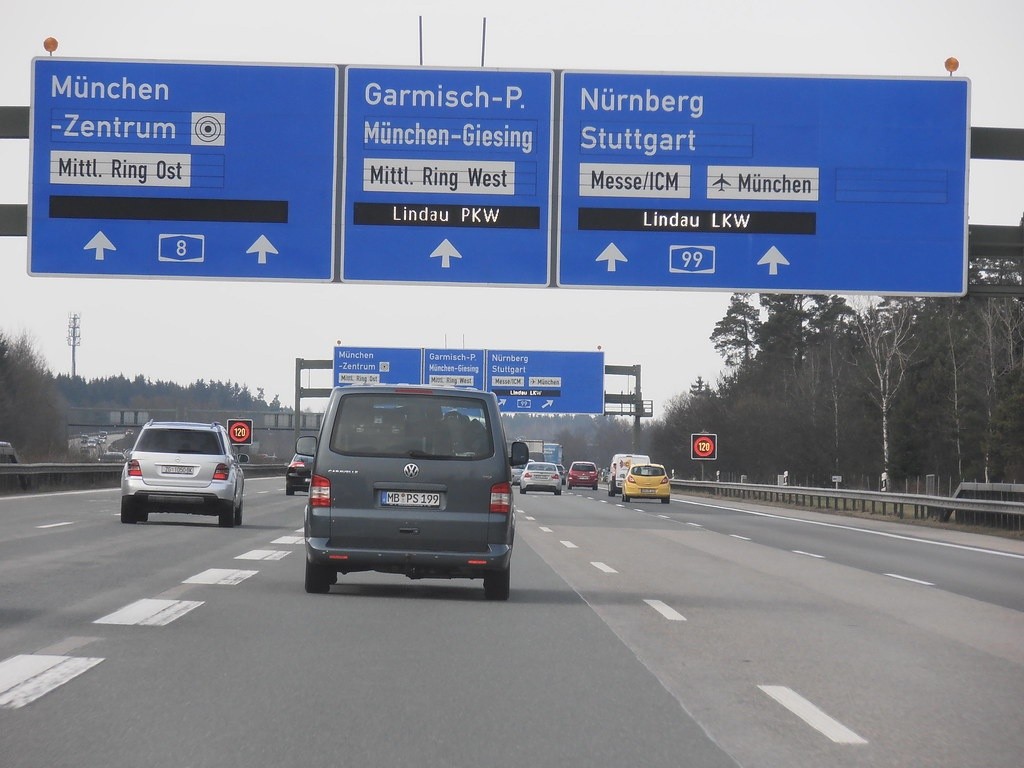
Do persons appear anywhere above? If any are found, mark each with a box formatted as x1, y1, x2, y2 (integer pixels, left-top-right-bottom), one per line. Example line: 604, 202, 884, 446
637, 467, 641, 473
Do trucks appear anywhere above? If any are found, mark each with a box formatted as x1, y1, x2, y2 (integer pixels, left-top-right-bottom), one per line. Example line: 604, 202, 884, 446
608, 453, 650, 497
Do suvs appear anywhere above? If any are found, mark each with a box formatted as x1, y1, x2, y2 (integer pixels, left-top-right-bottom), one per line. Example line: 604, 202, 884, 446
120, 419, 249, 528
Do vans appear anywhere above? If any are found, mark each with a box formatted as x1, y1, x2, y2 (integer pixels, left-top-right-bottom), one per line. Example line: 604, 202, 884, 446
294, 382, 529, 599
510, 459, 535, 485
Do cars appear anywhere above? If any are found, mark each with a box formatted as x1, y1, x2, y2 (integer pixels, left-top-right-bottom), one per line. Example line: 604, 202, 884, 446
516, 436, 526, 442
285, 452, 315, 495
563, 461, 598, 490
520, 462, 562, 495
622, 463, 670, 503
125, 429, 134, 436
88, 439, 97, 447
98, 452, 128, 463
80, 435, 89, 443
556, 464, 568, 485
98, 431, 107, 439
95, 438, 106, 444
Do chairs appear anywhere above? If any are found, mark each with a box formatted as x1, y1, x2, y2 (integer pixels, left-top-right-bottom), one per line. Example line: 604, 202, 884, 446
354, 406, 406, 449
653, 470, 661, 476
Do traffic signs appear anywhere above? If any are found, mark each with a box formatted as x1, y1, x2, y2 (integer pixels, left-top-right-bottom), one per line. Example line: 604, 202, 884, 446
332, 345, 606, 417
28, 55, 971, 301
